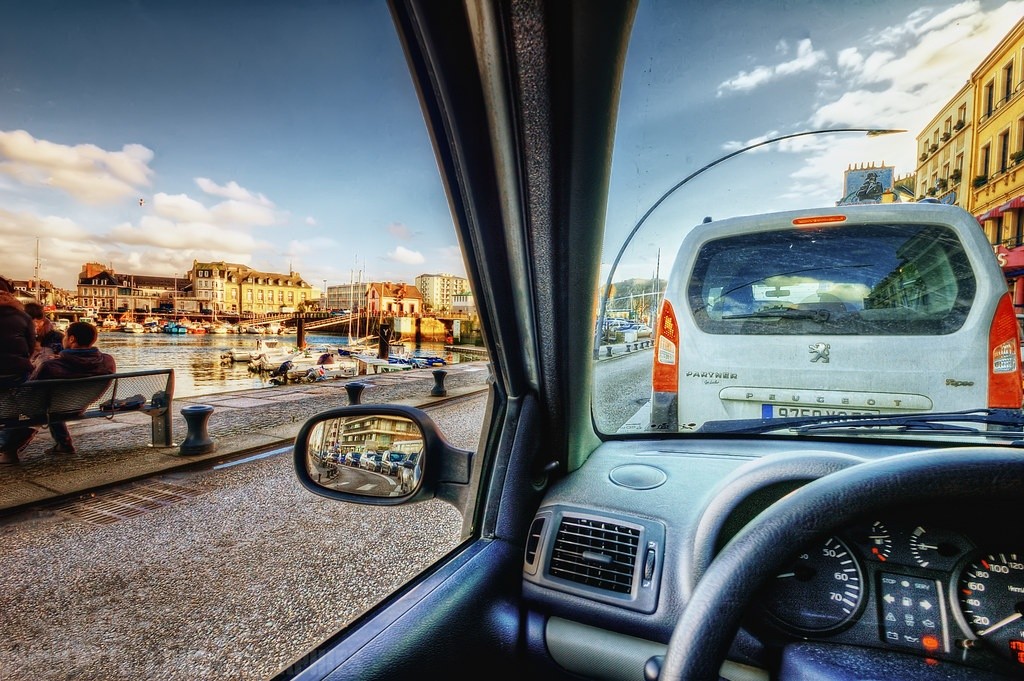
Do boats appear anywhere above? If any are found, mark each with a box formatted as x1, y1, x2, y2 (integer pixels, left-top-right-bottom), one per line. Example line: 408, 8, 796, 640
222, 339, 445, 385
56, 316, 287, 336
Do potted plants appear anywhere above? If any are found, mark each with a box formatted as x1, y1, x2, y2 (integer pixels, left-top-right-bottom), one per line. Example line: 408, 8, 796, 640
1010, 151, 1024, 164
953, 120, 964, 130
935, 178, 947, 188
926, 187, 935, 195
928, 144, 938, 153
972, 174, 987, 189
949, 168, 961, 185
915, 195, 925, 202
919, 153, 928, 161
940, 132, 951, 142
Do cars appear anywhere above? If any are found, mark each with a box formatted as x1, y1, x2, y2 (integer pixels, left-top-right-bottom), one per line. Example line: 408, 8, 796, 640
396, 448, 424, 494
603, 317, 616, 325
615, 318, 627, 323
337, 455, 345, 464
608, 321, 627, 332
621, 325, 652, 338
627, 320, 639, 326
369, 455, 382, 472
619, 323, 641, 331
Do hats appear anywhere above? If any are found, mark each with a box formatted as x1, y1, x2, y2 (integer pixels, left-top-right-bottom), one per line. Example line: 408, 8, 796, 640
865, 172, 880, 180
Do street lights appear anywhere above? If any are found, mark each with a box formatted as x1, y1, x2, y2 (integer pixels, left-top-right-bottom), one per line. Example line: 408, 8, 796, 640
595, 127, 909, 356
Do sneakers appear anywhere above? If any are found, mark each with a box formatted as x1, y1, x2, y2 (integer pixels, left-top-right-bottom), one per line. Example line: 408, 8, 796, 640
45, 444, 77, 455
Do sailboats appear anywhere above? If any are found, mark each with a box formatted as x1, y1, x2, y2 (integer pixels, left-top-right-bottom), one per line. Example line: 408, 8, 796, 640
335, 266, 402, 354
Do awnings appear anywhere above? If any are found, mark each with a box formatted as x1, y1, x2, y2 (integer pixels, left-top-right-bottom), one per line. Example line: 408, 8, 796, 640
976, 194, 1024, 225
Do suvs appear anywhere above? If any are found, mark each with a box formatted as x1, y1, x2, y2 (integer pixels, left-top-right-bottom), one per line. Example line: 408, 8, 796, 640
344, 452, 361, 467
323, 452, 339, 462
381, 450, 408, 476
359, 452, 378, 470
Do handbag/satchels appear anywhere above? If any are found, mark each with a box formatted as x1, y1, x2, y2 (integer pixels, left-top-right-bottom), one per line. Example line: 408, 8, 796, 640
99, 394, 146, 420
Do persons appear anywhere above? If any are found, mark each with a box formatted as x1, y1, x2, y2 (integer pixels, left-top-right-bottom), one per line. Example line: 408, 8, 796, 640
0, 275, 116, 467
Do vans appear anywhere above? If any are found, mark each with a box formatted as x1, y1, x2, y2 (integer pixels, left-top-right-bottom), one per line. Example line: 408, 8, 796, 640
650, 203, 1024, 447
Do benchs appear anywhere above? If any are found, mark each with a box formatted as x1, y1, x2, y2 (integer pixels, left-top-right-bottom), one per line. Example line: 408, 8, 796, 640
0, 369, 174, 447
